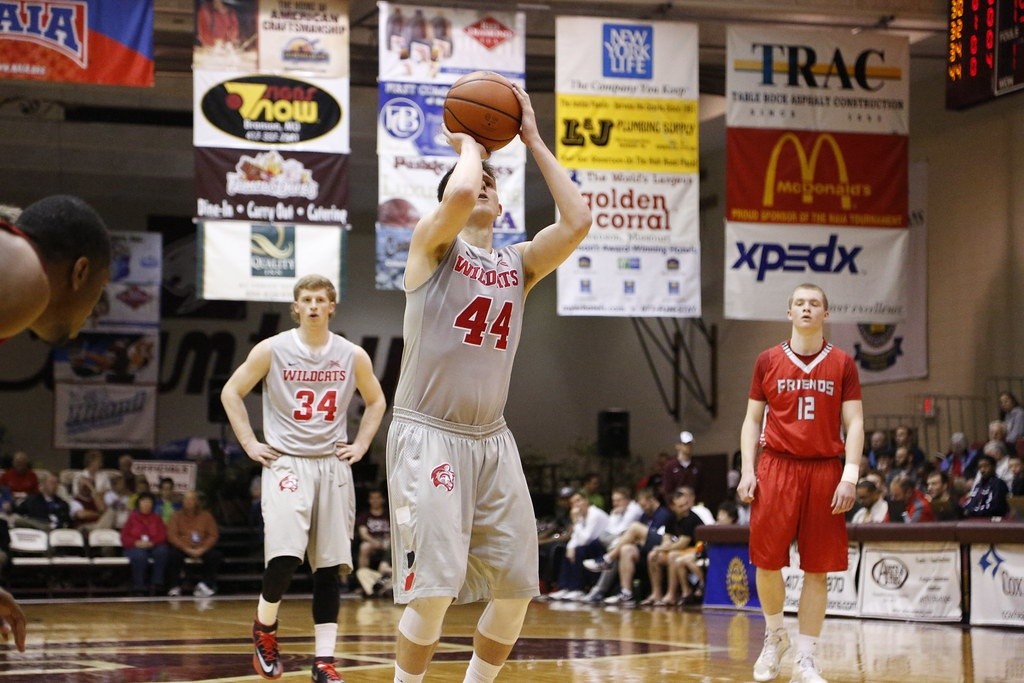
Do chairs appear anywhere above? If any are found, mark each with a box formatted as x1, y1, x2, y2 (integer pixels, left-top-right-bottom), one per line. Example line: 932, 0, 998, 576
0, 465, 205, 568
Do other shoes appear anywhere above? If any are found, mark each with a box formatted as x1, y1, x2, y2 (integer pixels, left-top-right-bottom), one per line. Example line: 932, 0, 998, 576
677, 594, 702, 605
641, 600, 670, 606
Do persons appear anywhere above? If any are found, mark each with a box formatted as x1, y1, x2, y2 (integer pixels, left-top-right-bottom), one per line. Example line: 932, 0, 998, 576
0, 451, 394, 597
737, 283, 864, 683
0, 194, 114, 652
536, 432, 750, 608
851, 391, 1024, 526
221, 275, 387, 683
386, 83, 593, 683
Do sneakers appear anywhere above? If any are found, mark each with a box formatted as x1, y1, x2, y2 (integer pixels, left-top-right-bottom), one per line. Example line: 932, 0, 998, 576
562, 591, 584, 600
580, 588, 604, 603
583, 556, 614, 572
312, 662, 344, 683
548, 589, 566, 600
792, 654, 828, 683
251, 618, 282, 680
168, 587, 181, 596
193, 584, 215, 595
603, 593, 636, 605
753, 633, 790, 682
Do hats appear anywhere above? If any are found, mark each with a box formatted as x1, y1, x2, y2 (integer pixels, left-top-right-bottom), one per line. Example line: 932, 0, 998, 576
678, 431, 695, 444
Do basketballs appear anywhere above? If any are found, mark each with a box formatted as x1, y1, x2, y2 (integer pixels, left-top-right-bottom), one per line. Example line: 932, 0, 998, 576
443, 71, 522, 153
